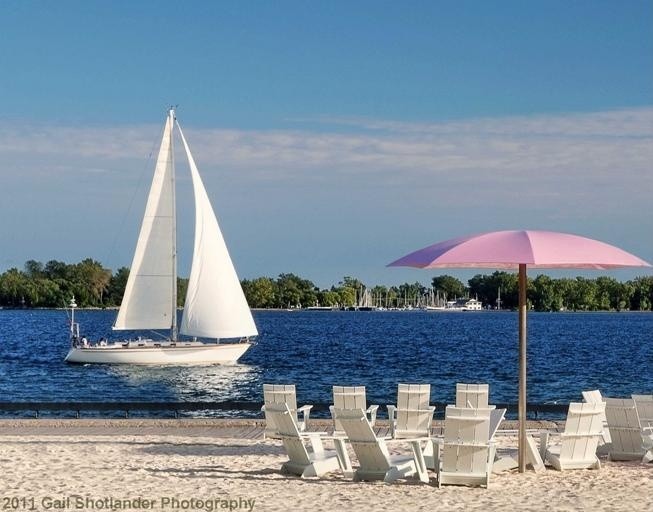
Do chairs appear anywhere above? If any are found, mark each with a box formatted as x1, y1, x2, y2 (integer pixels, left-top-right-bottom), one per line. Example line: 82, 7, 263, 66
259, 380, 509, 490
525, 388, 653, 474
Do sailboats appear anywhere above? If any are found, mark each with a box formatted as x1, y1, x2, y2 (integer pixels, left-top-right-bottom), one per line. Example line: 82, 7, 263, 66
63, 105, 259, 368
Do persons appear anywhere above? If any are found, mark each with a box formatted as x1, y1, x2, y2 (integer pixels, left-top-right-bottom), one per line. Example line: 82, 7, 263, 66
99, 337, 108, 346
80, 335, 87, 345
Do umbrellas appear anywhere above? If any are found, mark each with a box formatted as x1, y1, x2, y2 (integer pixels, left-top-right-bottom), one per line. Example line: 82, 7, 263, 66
385, 230, 653, 475
